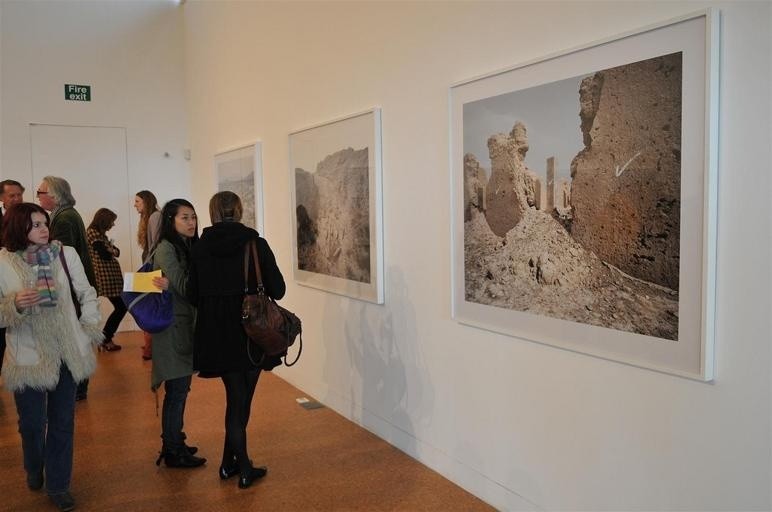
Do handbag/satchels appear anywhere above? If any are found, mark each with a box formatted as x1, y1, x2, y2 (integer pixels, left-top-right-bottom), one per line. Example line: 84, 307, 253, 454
238, 290, 304, 369
118, 259, 177, 336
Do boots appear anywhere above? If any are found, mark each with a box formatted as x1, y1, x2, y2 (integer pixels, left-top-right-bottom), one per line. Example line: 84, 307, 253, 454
140, 328, 154, 360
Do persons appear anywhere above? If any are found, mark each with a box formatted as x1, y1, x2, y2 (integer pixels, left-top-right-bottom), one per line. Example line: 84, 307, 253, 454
84, 206, 132, 356
135, 187, 164, 362
36, 172, 91, 407
142, 195, 209, 470
0, 202, 106, 512
0, 178, 27, 381
183, 188, 286, 495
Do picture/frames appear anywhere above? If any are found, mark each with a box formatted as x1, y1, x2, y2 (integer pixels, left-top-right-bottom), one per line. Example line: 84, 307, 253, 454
211, 138, 266, 241
285, 103, 388, 309
443, 3, 718, 386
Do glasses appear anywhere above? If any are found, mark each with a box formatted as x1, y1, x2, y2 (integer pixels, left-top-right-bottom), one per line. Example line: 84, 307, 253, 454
36, 189, 50, 197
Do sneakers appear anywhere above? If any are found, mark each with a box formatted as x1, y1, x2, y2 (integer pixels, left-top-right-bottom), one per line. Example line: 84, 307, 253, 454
102, 338, 123, 352
75, 390, 89, 403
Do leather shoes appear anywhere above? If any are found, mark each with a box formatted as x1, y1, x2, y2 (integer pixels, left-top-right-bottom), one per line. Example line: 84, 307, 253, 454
159, 442, 207, 470
45, 489, 76, 512
27, 459, 44, 492
238, 465, 270, 491
219, 461, 252, 479
